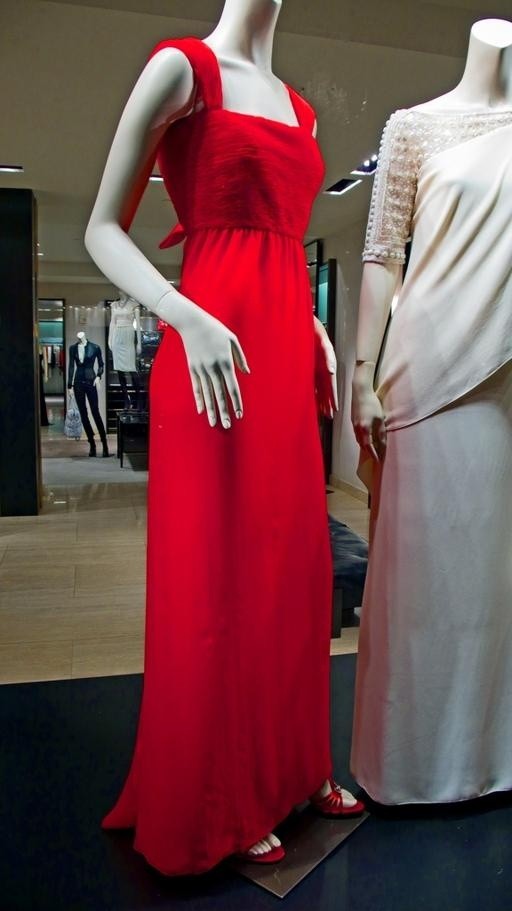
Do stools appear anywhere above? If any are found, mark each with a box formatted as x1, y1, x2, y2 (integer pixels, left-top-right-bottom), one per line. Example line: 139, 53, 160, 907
328, 512, 373, 638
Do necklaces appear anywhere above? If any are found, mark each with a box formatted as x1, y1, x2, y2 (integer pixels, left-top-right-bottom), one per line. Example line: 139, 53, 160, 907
118, 296, 130, 309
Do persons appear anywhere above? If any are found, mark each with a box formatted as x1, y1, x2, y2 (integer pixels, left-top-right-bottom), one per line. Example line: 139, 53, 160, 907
107, 289, 145, 413
346, 10, 510, 823
67, 332, 110, 459
80, 1, 371, 881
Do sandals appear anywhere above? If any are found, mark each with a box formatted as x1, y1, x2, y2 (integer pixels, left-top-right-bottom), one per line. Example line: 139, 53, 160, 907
244, 833, 286, 863
311, 777, 366, 818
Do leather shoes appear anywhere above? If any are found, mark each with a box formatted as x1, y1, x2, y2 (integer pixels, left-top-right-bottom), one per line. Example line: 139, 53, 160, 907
89, 445, 109, 457
